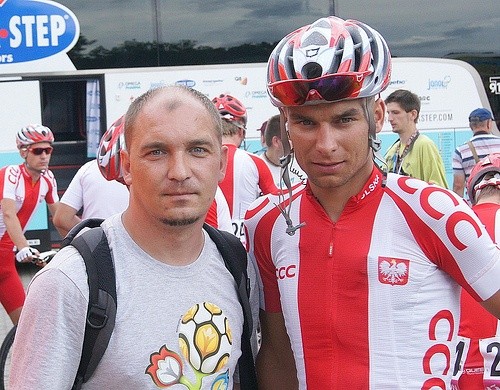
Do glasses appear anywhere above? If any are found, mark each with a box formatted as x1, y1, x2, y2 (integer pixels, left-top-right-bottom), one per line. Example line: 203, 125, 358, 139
21, 146, 53, 154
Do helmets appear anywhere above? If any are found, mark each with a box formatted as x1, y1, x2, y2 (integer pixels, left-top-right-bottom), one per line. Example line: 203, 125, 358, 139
212, 94, 247, 124
16, 124, 54, 149
266, 15, 392, 106
96, 114, 130, 186
467, 151, 500, 204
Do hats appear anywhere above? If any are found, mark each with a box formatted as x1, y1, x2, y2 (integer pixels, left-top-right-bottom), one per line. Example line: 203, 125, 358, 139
257, 121, 267, 134
468, 108, 496, 121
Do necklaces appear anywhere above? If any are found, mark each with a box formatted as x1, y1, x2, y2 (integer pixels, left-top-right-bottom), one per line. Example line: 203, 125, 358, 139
264, 151, 280, 167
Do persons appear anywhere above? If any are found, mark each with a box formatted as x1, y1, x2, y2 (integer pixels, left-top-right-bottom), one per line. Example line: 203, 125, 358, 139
210, 95, 278, 250
380, 89, 447, 190
452, 152, 500, 390
4, 86, 260, 389
53, 157, 129, 233
242, 17, 500, 390
98, 110, 233, 237
0, 124, 70, 326
260, 115, 307, 188
452, 108, 500, 199
